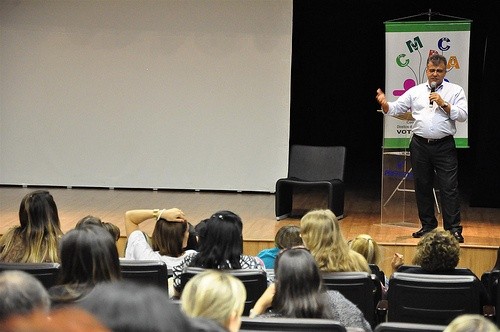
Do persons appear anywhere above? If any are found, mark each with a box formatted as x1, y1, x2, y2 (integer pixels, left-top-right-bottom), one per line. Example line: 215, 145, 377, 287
172, 211, 266, 301
376, 54, 468, 244
48, 215, 120, 311
279, 209, 373, 273
439, 246, 500, 332
255, 227, 308, 270
124, 208, 189, 298
347, 235, 391, 325
0, 190, 65, 265
1, 266, 248, 331
250, 248, 373, 332
389, 230, 475, 275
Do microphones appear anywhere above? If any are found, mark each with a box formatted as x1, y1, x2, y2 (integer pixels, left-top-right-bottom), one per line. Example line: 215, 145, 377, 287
429, 81, 437, 107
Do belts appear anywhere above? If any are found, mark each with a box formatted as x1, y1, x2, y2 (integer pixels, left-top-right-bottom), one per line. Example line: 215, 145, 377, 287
413, 133, 453, 144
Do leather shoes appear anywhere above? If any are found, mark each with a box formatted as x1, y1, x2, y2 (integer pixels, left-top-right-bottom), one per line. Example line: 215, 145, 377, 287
452, 231, 464, 242
412, 228, 429, 237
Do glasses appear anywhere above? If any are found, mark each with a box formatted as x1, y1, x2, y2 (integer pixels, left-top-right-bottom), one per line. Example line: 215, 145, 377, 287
428, 66, 446, 74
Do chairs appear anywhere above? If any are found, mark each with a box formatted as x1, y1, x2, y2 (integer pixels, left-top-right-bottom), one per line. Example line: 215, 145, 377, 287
321, 271, 388, 321
0, 262, 60, 288
182, 268, 269, 314
119, 259, 169, 299
377, 273, 495, 322
275, 145, 347, 221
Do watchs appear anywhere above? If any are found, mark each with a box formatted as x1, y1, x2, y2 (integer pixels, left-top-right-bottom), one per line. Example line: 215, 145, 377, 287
441, 101, 449, 109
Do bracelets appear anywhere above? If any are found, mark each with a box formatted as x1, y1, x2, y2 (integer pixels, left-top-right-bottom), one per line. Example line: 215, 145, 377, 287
153, 208, 165, 221
